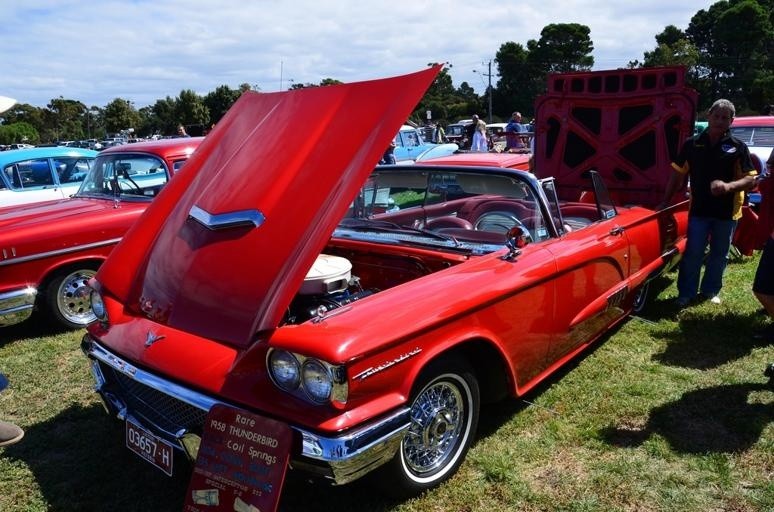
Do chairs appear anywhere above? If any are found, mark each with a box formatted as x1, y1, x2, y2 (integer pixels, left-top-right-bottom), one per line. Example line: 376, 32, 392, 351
459, 199, 604, 250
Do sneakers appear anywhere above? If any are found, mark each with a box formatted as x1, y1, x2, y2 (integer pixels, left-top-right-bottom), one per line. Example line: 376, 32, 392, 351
0, 421, 25, 448
676, 291, 720, 306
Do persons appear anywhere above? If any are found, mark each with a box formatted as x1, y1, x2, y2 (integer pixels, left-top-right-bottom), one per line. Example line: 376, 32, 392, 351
431, 119, 447, 144
93, 141, 102, 150
506, 111, 535, 150
378, 139, 396, 165
467, 114, 489, 152
671, 98, 774, 378
177, 124, 191, 137
128, 132, 137, 143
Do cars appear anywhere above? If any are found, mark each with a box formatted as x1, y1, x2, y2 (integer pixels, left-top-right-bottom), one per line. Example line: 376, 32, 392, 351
0, 137, 206, 327
